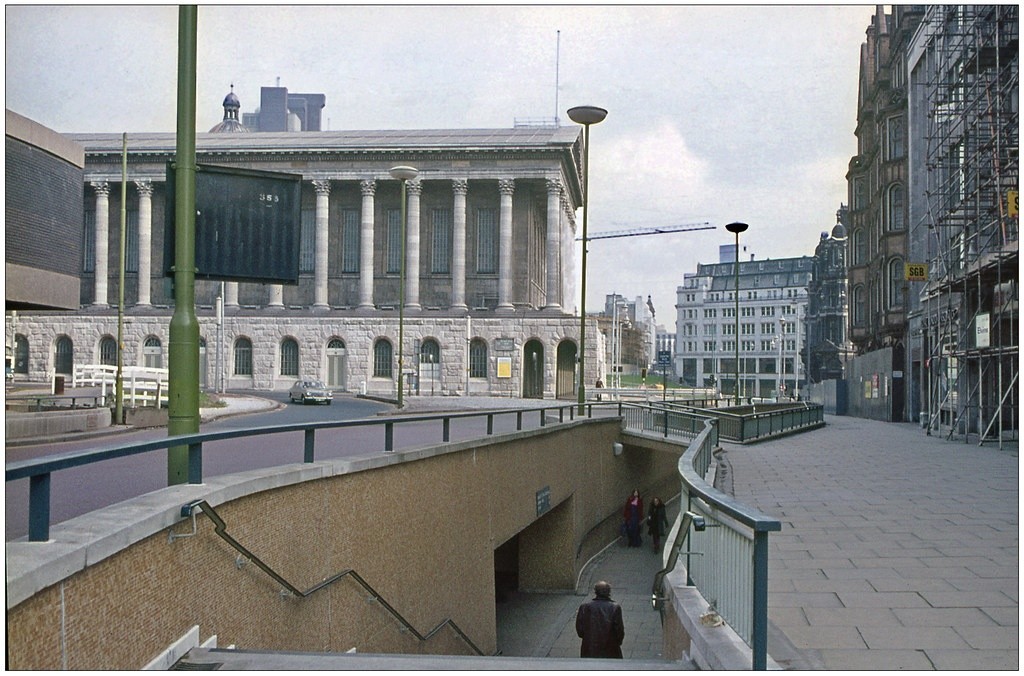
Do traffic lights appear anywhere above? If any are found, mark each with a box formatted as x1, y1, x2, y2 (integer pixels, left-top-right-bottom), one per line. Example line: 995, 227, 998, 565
641, 368, 646, 381
619, 366, 623, 373
613, 366, 616, 372
710, 375, 714, 385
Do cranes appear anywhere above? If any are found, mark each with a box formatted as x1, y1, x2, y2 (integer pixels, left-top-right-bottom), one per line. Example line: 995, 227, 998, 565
574, 222, 717, 242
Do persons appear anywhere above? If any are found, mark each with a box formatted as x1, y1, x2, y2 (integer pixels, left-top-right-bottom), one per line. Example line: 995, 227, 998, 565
719, 391, 722, 399
596, 377, 604, 402
314, 383, 322, 389
779, 386, 785, 397
576, 581, 625, 659
647, 497, 669, 555
626, 488, 643, 519
624, 496, 641, 547
309, 382, 313, 387
303, 382, 308, 388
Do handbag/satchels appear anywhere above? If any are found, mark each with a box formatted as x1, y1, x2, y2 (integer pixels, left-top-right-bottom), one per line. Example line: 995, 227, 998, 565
621, 525, 626, 537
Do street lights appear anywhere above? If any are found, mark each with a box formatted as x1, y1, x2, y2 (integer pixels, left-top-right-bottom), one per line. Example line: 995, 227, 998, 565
790, 296, 800, 401
712, 348, 723, 407
610, 292, 635, 401
387, 166, 421, 408
770, 314, 787, 397
566, 106, 608, 416
725, 223, 750, 405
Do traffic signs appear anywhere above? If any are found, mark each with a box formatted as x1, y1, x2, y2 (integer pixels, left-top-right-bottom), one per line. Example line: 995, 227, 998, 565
657, 349, 672, 367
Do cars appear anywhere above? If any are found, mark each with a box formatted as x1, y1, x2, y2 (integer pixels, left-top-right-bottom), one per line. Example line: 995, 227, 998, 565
286, 380, 333, 404
